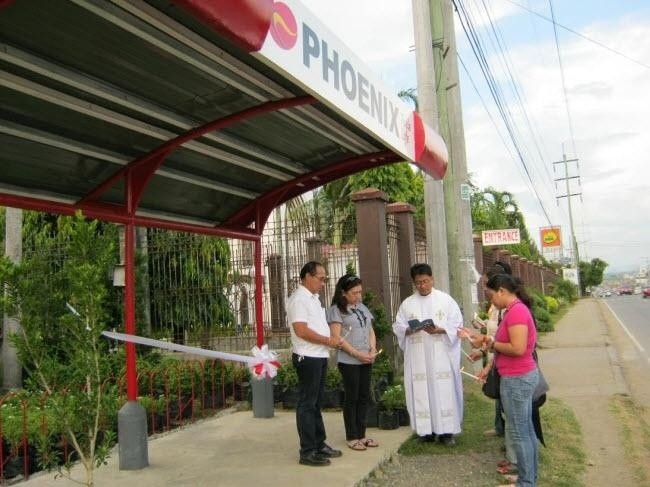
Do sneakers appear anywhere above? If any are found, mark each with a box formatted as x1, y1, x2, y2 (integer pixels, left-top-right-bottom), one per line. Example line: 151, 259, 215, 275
439, 434, 457, 448
497, 456, 523, 487
419, 432, 437, 443
482, 426, 504, 437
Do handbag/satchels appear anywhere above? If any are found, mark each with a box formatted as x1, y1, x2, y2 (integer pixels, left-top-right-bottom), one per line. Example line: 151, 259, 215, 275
482, 364, 506, 399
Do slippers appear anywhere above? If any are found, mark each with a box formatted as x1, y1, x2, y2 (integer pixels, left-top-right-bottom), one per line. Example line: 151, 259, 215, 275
344, 438, 368, 450
360, 437, 379, 447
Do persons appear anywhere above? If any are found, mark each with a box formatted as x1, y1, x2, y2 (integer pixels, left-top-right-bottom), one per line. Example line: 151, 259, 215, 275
391, 262, 465, 450
456, 261, 552, 487
324, 273, 380, 452
285, 260, 346, 467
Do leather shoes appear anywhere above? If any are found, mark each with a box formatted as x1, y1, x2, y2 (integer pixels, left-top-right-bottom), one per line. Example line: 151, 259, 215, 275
321, 445, 343, 458
299, 448, 331, 467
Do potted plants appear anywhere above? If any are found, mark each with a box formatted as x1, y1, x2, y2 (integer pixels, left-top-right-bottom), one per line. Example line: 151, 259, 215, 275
236, 350, 411, 432
1, 342, 235, 483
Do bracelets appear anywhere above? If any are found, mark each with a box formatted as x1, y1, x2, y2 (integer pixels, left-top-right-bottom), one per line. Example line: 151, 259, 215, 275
349, 349, 356, 356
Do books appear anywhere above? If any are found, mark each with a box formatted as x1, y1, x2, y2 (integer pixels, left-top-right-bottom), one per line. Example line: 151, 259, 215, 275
408, 318, 435, 332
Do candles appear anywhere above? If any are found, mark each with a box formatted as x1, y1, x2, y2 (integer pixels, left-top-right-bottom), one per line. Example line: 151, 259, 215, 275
456, 311, 486, 384
341, 325, 353, 343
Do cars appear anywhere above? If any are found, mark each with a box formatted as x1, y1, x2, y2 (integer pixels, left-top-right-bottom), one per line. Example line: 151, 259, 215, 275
594, 281, 650, 298
